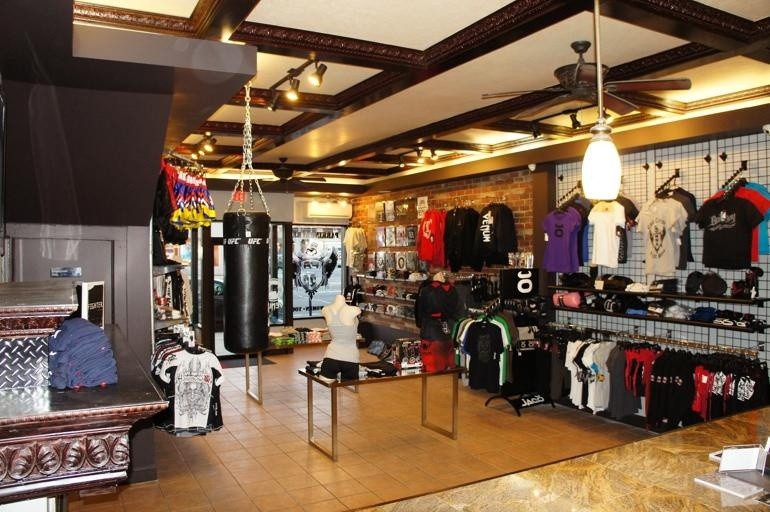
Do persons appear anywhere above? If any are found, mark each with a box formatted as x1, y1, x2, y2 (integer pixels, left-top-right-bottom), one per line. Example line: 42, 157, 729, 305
319, 294, 362, 382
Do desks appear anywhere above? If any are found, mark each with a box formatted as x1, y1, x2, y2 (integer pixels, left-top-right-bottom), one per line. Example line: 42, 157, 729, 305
0, 280, 168, 512
245, 330, 466, 462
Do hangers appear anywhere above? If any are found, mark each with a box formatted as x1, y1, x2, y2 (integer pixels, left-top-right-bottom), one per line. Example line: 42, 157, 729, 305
462, 298, 506, 329
349, 218, 362, 229
569, 325, 767, 374
552, 167, 757, 218
433, 272, 447, 282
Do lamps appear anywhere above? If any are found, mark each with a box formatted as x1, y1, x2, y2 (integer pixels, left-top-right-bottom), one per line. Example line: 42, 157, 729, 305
186, 135, 217, 164
398, 142, 438, 169
580, 0, 623, 202
263, 55, 330, 113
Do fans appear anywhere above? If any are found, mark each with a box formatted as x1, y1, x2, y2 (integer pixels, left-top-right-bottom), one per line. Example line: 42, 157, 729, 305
481, 40, 692, 117
